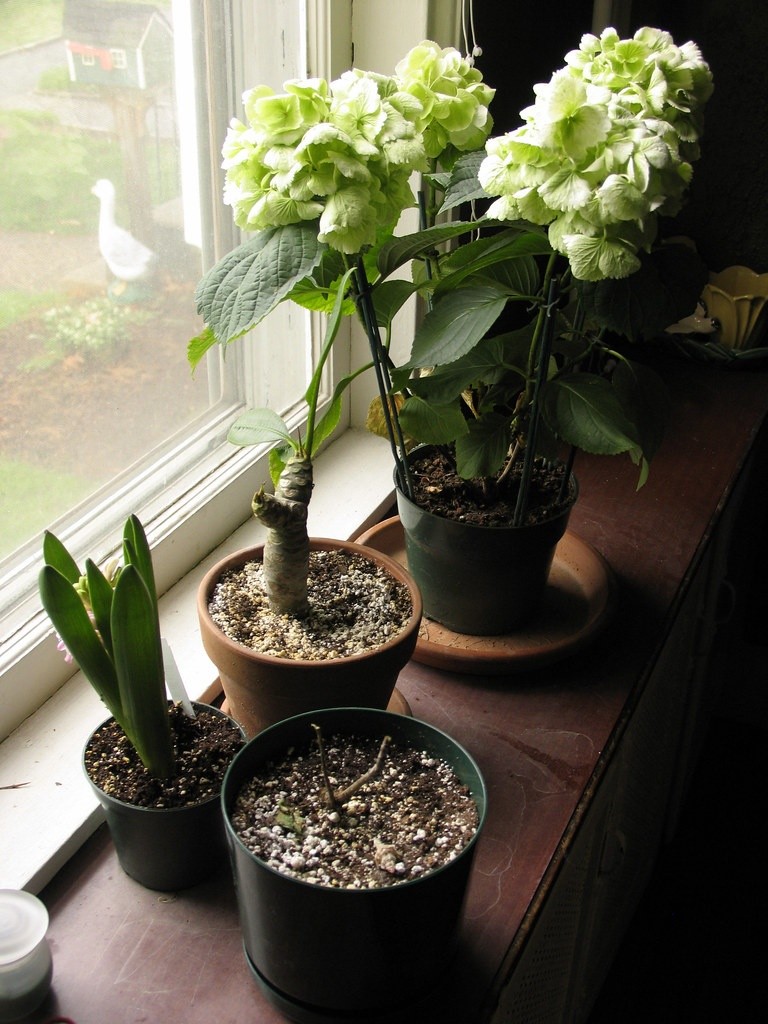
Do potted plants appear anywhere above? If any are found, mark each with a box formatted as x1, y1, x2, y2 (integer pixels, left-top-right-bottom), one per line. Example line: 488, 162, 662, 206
196, 265, 422, 739
37, 513, 248, 893
220, 705, 488, 1024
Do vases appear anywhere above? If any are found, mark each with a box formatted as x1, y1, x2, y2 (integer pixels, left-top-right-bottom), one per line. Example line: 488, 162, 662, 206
700, 265, 768, 350
394, 442, 581, 639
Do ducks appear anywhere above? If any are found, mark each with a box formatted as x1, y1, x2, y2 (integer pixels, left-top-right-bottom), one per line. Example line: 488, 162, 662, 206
91, 178, 161, 285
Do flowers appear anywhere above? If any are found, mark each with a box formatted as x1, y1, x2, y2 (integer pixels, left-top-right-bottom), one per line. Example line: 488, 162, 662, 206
219, 27, 717, 524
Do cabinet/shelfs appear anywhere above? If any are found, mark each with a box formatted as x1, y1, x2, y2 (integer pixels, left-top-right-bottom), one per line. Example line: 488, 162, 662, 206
0, 357, 768, 1024
